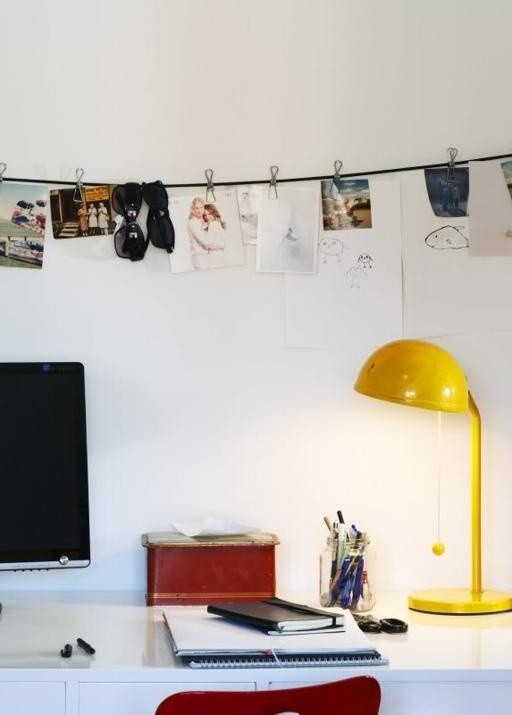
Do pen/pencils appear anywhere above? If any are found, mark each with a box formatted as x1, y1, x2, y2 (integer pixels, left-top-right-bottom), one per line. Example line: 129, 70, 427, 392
77, 638, 94, 654
325, 510, 372, 609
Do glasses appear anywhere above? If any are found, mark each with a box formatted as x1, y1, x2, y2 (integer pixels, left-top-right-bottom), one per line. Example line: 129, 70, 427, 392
111, 183, 149, 260
144, 180, 175, 253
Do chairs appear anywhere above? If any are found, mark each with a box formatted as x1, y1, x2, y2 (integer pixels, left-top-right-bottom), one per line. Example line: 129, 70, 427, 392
154, 675, 381, 715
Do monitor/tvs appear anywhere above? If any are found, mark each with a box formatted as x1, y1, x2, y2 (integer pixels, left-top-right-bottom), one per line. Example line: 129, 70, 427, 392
0, 361, 91, 613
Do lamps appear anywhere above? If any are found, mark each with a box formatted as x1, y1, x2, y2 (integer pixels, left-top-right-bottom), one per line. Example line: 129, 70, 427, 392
354, 339, 512, 616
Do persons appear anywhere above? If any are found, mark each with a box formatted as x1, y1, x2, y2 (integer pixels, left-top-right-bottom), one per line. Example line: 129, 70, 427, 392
201, 203, 227, 269
205, 596, 346, 631
255, 624, 347, 635
97, 201, 109, 235
439, 183, 461, 211
77, 203, 89, 237
186, 196, 226, 270
88, 202, 98, 236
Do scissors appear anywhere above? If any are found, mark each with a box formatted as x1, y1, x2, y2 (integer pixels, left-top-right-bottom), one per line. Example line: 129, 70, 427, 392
352, 614, 407, 634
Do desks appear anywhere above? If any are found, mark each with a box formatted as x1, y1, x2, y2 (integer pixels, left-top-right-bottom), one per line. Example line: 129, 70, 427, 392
0, 591, 512, 714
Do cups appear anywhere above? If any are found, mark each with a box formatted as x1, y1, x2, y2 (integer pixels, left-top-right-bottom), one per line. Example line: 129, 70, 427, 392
319, 535, 378, 611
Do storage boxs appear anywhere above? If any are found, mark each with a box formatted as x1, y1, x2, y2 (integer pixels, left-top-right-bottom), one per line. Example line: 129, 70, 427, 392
140, 532, 281, 605
